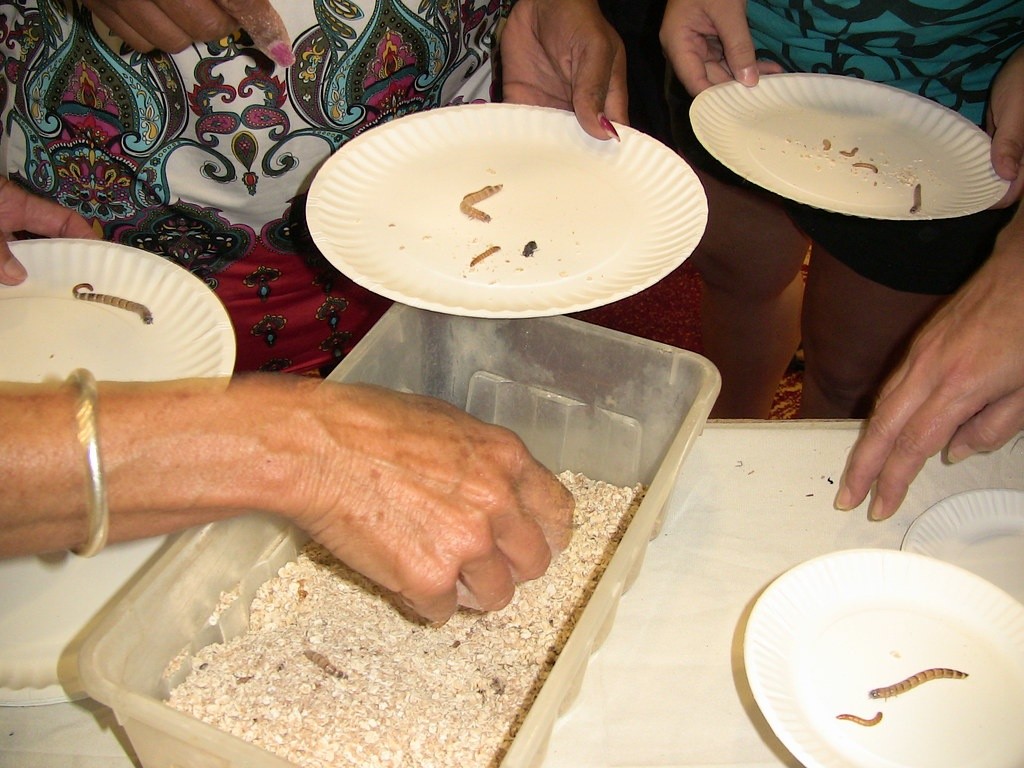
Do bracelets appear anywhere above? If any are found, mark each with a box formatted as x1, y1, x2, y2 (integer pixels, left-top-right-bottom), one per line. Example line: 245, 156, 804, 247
61, 367, 108, 558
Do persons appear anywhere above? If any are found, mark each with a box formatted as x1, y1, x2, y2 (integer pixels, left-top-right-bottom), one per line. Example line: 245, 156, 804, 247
0, 0, 1024, 629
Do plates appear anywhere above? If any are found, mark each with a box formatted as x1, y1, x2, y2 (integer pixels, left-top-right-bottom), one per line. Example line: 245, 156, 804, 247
689, 72, 1012, 220
306, 103, 708, 320
901, 488, 1024, 604
0, 536, 168, 709
0, 237, 236, 382
743, 547, 1024, 768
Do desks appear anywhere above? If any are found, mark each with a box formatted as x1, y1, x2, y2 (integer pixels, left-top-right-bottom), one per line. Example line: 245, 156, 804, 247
0, 419, 1024, 768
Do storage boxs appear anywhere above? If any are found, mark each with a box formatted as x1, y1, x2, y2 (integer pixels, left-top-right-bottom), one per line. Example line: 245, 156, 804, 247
75, 304, 724, 768
